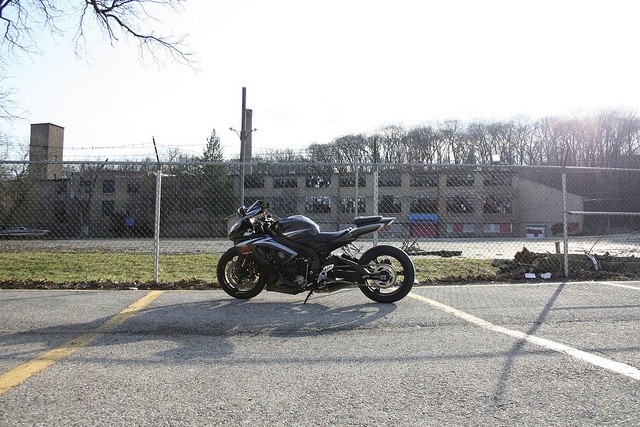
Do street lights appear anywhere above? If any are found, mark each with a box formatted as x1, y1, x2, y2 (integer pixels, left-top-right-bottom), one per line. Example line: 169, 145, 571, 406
229, 126, 259, 207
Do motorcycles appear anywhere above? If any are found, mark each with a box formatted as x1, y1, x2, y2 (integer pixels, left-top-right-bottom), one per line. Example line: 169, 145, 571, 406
216, 199, 416, 305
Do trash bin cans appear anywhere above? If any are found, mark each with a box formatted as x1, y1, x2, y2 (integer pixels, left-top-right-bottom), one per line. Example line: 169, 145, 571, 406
409, 213, 438, 237
